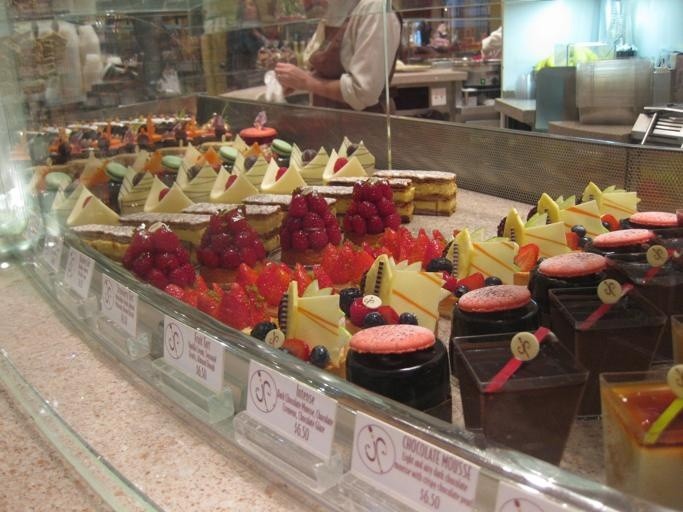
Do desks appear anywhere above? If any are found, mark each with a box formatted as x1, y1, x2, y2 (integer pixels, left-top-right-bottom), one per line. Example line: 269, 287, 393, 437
491, 96, 548, 134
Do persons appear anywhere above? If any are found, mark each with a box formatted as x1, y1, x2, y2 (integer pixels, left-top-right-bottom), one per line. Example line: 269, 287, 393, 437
275, 0, 406, 114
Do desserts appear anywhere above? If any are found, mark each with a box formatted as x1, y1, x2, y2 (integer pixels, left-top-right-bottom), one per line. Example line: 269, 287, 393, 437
8, 107, 678, 413
